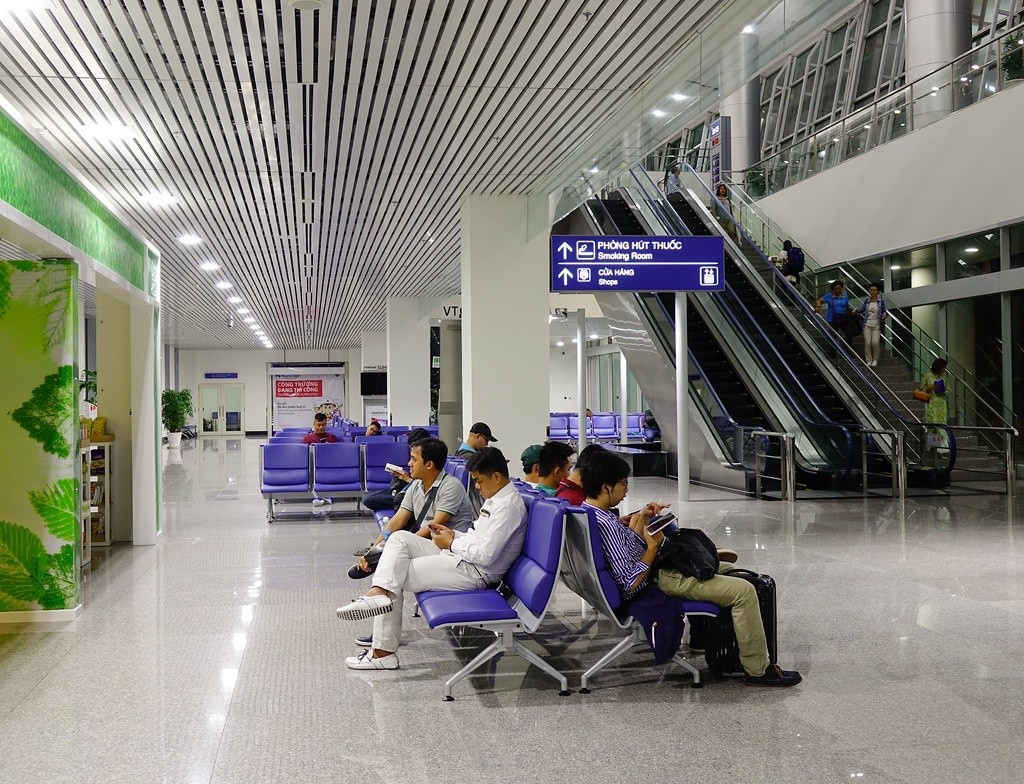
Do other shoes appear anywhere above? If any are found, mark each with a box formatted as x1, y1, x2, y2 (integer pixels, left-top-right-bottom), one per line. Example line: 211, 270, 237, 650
353, 548, 370, 556
872, 360, 878, 366
937, 454, 948, 459
313, 500, 324, 506
325, 498, 332, 504
867, 361, 872, 366
927, 446, 930, 451
716, 548, 738, 563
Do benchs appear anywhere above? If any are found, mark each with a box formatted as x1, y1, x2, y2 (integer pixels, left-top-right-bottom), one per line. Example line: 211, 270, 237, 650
181, 423, 198, 439
260, 412, 720, 702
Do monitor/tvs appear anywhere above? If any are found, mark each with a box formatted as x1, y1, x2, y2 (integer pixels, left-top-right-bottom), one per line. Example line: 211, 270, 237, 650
360, 372, 387, 396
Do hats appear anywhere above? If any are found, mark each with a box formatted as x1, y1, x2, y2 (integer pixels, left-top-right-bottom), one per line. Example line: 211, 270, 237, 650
521, 445, 543, 465
470, 422, 498, 442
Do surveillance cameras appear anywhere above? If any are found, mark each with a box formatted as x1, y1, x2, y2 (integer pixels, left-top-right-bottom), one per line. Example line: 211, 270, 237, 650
227, 320, 233, 328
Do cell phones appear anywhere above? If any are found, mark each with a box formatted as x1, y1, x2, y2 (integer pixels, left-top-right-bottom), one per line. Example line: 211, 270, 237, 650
320, 438, 327, 443
426, 525, 440, 535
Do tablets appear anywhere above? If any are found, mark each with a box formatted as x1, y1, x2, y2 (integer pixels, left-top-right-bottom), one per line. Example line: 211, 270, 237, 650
646, 511, 679, 536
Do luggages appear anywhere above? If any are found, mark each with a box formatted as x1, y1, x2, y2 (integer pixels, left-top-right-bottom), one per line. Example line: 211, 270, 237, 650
703, 569, 777, 675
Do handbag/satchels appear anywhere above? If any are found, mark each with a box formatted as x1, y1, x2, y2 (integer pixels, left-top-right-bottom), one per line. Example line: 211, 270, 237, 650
388, 476, 409, 496
844, 317, 864, 338
653, 528, 721, 581
914, 388, 930, 402
833, 313, 841, 326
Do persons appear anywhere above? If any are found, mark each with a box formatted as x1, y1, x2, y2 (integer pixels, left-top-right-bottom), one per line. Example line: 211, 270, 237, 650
454, 422, 519, 482
523, 440, 802, 688
711, 184, 735, 242
585, 409, 592, 428
913, 358, 950, 461
642, 409, 662, 442
668, 167, 681, 195
302, 413, 340, 507
780, 240, 805, 291
335, 446, 528, 671
346, 438, 473, 579
352, 421, 382, 444
814, 280, 857, 359
856, 284, 888, 367
362, 428, 431, 510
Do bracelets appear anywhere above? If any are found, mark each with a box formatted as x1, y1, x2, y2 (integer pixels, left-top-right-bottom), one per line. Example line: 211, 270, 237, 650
449, 539, 452, 552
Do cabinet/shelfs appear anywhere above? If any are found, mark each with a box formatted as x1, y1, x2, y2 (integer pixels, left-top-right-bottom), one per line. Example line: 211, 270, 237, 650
78, 442, 114, 567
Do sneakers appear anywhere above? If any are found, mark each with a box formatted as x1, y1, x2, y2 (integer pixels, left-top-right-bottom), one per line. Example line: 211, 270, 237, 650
347, 564, 375, 579
335, 595, 394, 621
743, 665, 802, 687
354, 634, 373, 646
675, 639, 704, 654
345, 649, 400, 669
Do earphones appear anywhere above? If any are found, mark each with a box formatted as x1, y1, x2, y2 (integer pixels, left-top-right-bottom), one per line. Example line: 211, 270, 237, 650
604, 487, 610, 493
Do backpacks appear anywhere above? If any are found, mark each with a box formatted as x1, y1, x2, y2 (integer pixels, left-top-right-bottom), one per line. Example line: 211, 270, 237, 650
784, 247, 805, 271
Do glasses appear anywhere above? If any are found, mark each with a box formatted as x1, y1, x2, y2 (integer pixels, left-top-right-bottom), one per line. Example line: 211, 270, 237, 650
475, 432, 488, 446
618, 481, 628, 489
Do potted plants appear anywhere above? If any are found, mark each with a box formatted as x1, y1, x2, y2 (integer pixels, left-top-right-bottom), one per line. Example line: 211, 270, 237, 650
741, 164, 776, 202
162, 389, 195, 450
